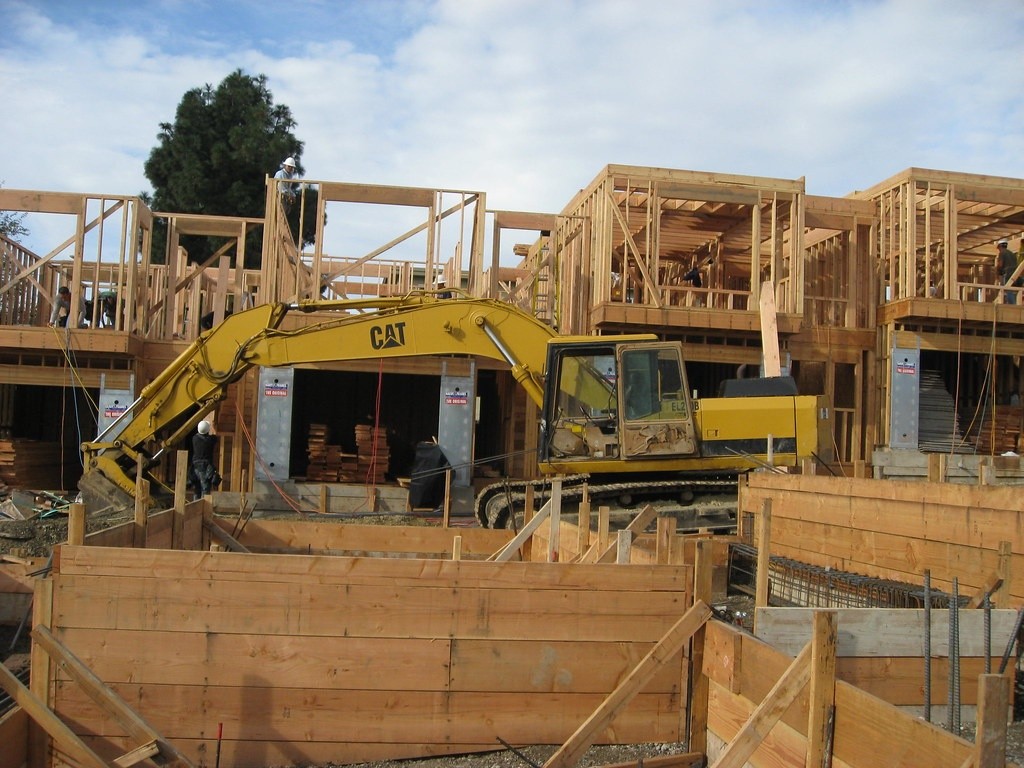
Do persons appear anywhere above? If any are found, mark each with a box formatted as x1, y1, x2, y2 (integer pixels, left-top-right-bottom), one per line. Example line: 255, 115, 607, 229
104, 295, 116, 326
273, 157, 296, 215
190, 421, 218, 500
48, 287, 88, 328
432, 275, 452, 299
996, 239, 1021, 305
624, 373, 652, 419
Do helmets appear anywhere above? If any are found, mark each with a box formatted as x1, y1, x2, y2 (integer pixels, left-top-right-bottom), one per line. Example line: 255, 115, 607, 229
197, 420, 211, 434
996, 239, 1008, 249
282, 157, 296, 167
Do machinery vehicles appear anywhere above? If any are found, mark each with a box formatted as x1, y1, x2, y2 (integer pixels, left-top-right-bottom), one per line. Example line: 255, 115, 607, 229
77, 287, 831, 537
